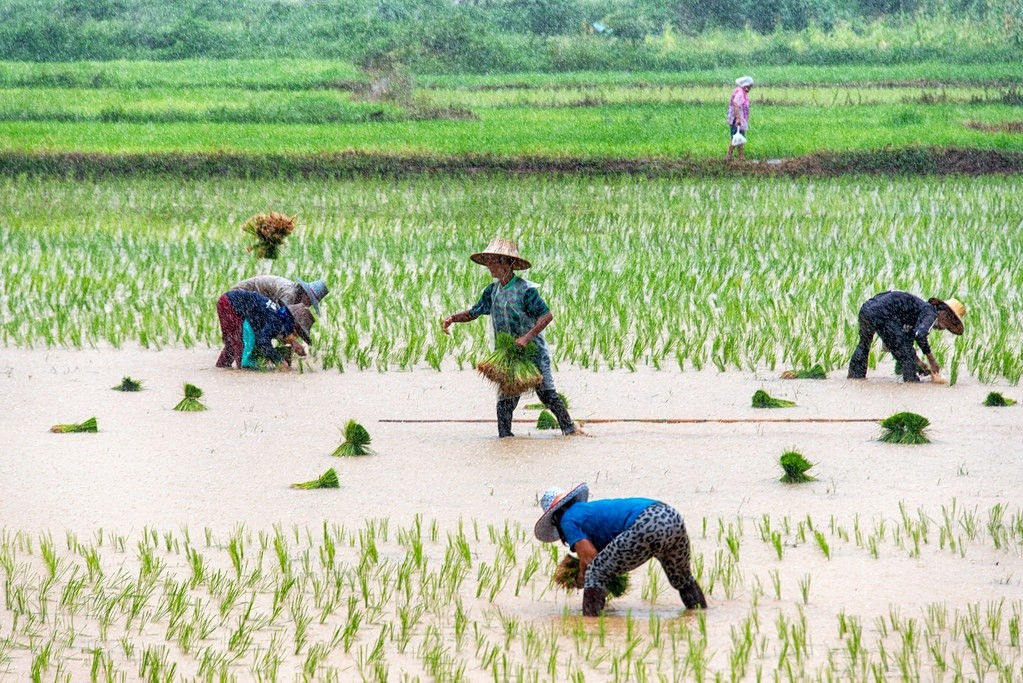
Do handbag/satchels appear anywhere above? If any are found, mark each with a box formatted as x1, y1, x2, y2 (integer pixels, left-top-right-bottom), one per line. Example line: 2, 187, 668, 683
731, 126, 746, 147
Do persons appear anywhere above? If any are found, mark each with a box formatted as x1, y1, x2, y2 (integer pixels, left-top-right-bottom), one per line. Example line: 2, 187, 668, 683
216, 290, 315, 370
229, 275, 329, 367
441, 239, 574, 437
534, 482, 706, 616
727, 77, 754, 160
846, 291, 966, 383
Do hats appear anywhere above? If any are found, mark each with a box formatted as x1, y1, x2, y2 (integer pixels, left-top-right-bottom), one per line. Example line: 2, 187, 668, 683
296, 279, 328, 317
535, 481, 589, 543
736, 76, 754, 88
928, 297, 966, 336
469, 239, 532, 270
277, 298, 316, 347
